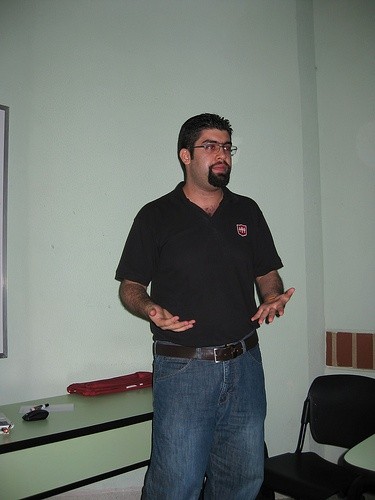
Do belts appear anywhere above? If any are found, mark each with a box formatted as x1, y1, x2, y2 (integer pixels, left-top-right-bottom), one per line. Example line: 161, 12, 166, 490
153, 330, 258, 364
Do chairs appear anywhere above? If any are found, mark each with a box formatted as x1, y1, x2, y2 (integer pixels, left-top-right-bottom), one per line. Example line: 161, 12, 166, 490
267, 371, 375, 500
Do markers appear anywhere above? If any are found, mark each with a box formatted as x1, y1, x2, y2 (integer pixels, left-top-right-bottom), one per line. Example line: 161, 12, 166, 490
30, 403, 49, 411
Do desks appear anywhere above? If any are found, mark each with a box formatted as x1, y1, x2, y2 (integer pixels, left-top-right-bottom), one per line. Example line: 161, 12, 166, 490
339, 433, 375, 478
0, 382, 163, 500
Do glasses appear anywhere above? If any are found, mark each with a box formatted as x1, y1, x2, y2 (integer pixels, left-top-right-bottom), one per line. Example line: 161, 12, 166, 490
186, 142, 238, 156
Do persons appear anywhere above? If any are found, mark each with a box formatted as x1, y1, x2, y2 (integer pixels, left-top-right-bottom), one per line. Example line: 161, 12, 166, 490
113, 112, 297, 500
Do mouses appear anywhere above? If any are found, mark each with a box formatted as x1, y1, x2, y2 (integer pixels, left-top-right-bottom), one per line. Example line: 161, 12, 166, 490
23, 410, 50, 422
